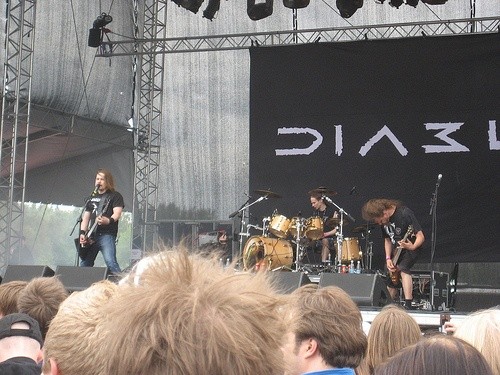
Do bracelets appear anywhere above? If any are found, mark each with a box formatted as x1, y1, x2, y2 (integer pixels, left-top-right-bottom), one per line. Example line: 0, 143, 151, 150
80, 230, 85, 234
385, 257, 390, 260
110, 218, 114, 224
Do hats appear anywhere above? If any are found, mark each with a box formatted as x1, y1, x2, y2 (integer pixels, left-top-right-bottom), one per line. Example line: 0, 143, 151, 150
0, 313, 44, 350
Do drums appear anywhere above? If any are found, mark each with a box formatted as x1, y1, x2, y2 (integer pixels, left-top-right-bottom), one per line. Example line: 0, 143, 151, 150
268, 214, 291, 239
288, 216, 308, 238
306, 216, 323, 240
333, 236, 362, 263
242, 235, 293, 274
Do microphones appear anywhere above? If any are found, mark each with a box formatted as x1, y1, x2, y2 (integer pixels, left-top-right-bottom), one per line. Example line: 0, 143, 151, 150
92, 184, 100, 197
436, 174, 443, 187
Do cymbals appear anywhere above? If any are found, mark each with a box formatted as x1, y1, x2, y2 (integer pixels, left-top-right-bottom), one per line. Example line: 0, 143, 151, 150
253, 189, 283, 199
325, 217, 349, 227
351, 223, 378, 233
307, 189, 338, 197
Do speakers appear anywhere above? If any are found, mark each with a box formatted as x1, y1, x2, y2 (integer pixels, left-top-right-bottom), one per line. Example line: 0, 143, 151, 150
1, 265, 55, 284
319, 273, 383, 308
54, 265, 108, 293
272, 272, 312, 295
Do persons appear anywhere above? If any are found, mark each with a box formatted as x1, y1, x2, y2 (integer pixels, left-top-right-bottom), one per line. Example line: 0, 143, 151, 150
42, 280, 118, 375
299, 192, 339, 261
356, 304, 423, 375
445, 306, 500, 375
362, 199, 424, 310
0, 281, 29, 318
0, 313, 44, 375
281, 284, 368, 375
99, 242, 291, 375
376, 334, 493, 375
79, 168, 121, 273
17, 273, 67, 338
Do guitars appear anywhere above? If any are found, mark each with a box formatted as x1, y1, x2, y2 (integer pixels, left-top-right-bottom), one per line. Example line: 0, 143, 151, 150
384, 224, 416, 288
73, 198, 112, 262
